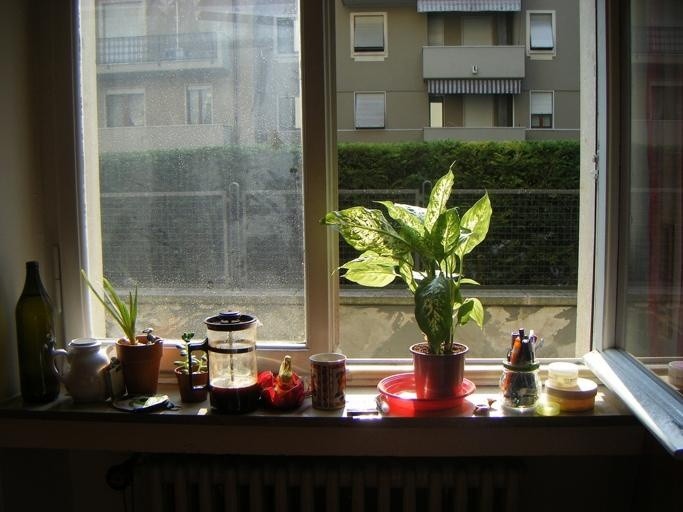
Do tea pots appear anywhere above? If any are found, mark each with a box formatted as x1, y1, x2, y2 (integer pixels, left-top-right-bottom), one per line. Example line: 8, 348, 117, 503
48, 334, 113, 404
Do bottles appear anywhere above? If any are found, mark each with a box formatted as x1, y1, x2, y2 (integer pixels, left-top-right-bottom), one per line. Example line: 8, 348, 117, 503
14, 260, 62, 405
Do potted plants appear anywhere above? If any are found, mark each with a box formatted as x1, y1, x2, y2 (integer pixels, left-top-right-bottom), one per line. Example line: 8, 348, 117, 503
320, 160, 494, 401
79, 268, 163, 396
173, 331, 209, 404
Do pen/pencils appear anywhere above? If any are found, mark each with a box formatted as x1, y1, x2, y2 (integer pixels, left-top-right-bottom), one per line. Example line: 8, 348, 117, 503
501, 328, 543, 390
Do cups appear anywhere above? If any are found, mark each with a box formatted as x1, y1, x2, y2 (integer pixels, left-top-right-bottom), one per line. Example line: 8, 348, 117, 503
498, 359, 544, 411
185, 310, 263, 413
308, 352, 347, 410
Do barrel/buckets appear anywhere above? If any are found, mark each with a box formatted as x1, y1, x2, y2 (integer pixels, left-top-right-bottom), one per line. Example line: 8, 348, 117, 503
409, 340, 469, 400
